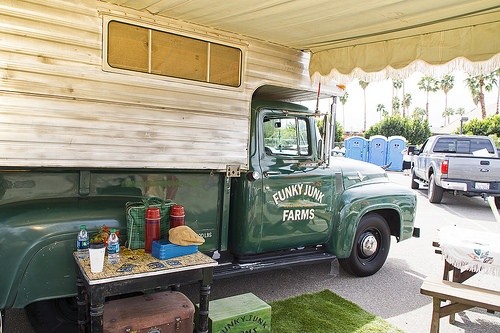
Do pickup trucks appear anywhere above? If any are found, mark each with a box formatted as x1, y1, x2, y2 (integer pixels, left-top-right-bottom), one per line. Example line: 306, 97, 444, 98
407, 134, 500, 211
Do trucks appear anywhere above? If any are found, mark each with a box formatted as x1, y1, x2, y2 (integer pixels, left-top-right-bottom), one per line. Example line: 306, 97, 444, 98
0, 0, 421, 333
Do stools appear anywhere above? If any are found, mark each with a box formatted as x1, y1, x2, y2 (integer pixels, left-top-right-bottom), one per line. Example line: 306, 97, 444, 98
420, 275, 500, 333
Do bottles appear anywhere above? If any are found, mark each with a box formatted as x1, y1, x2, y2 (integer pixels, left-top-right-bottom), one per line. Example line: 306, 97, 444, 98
107, 228, 120, 265
76, 225, 90, 261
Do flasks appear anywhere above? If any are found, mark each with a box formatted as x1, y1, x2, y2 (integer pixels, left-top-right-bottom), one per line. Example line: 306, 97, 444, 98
170, 205, 185, 228
145, 208, 161, 253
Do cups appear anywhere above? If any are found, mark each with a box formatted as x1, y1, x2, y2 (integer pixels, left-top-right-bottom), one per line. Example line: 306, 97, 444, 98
88, 242, 106, 274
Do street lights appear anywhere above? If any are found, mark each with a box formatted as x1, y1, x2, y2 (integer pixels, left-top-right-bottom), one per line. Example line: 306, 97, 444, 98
460, 117, 468, 134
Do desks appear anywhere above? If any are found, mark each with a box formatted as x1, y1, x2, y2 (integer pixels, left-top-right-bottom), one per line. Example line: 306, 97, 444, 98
432, 218, 500, 303
73, 245, 219, 333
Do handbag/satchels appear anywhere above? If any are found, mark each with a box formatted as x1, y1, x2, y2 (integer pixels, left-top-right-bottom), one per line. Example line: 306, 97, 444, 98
124, 196, 176, 251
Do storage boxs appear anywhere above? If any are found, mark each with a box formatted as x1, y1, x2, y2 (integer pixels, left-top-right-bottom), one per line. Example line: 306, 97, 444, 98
101, 290, 195, 333
196, 292, 272, 333
152, 239, 198, 260
